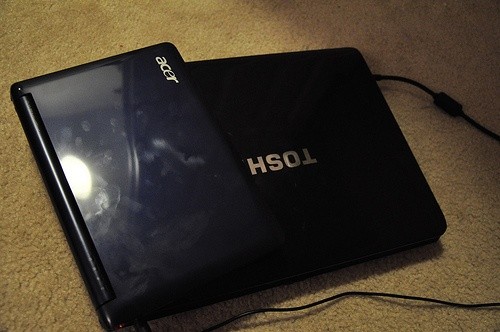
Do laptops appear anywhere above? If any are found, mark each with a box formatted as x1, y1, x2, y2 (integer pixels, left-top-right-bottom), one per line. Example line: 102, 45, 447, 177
105, 47, 447, 326
9, 40, 277, 329
